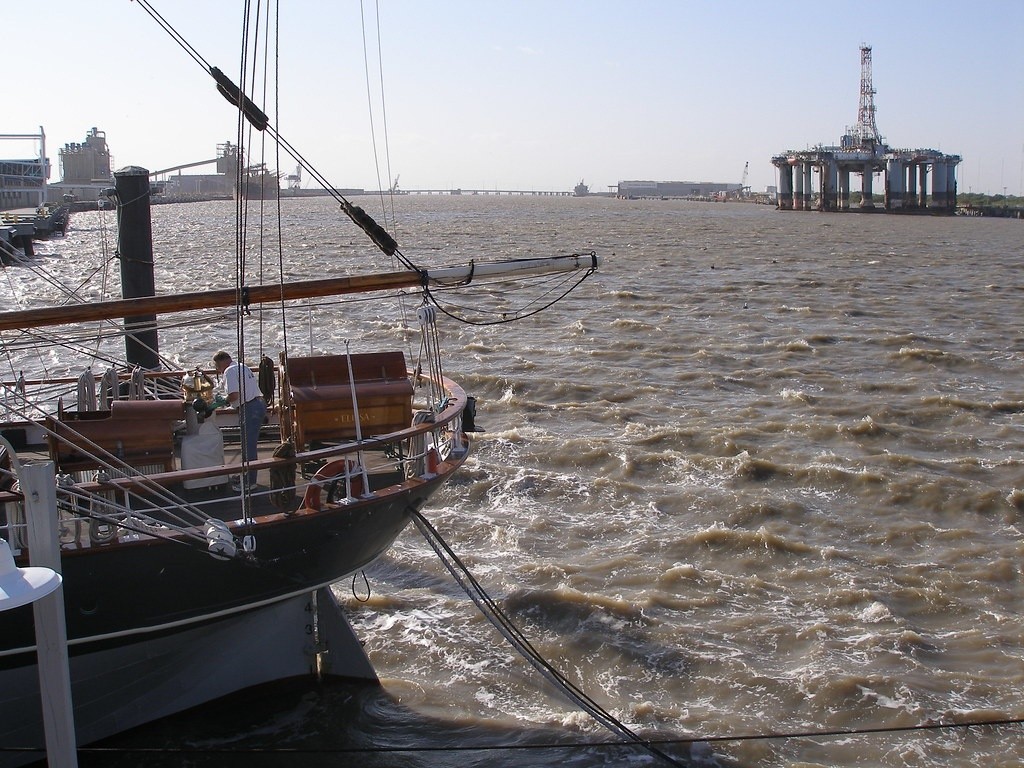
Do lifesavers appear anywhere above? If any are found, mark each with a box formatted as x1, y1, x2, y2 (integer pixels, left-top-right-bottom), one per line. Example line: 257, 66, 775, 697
304, 458, 364, 507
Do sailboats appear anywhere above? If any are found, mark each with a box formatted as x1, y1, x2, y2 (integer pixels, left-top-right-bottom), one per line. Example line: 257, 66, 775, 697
0, 0, 603, 768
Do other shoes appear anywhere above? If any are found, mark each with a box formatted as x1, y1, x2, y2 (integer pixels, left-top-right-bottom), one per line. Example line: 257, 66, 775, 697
231, 475, 248, 483
232, 482, 257, 491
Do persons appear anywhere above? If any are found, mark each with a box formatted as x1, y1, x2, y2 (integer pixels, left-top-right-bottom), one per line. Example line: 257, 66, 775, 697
210, 351, 267, 491
